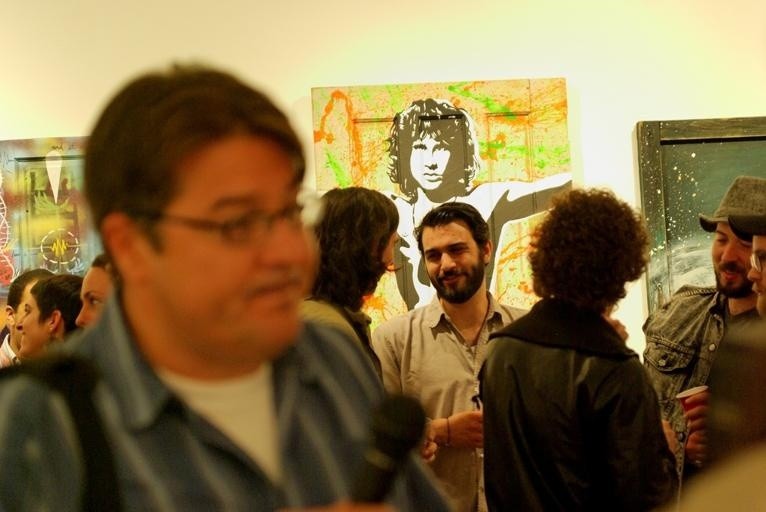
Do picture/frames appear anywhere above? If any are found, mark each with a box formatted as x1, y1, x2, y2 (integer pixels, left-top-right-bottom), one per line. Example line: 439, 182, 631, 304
630, 116, 766, 319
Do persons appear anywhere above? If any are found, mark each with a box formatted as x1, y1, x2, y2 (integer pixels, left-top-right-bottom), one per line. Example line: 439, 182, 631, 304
299, 186, 462, 512
643, 175, 766, 510
378, 100, 570, 313
2, 62, 463, 512
1, 254, 121, 367
369, 203, 533, 512
476, 186, 680, 511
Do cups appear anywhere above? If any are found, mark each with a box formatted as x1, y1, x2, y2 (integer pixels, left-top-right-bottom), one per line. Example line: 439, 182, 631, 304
674, 385, 711, 431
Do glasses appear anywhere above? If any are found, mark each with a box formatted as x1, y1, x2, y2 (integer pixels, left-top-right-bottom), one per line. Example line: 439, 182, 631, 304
750, 252, 766, 272
123, 202, 304, 249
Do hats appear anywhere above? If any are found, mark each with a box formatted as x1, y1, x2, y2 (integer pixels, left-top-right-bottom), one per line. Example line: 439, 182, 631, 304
698, 176, 766, 242
